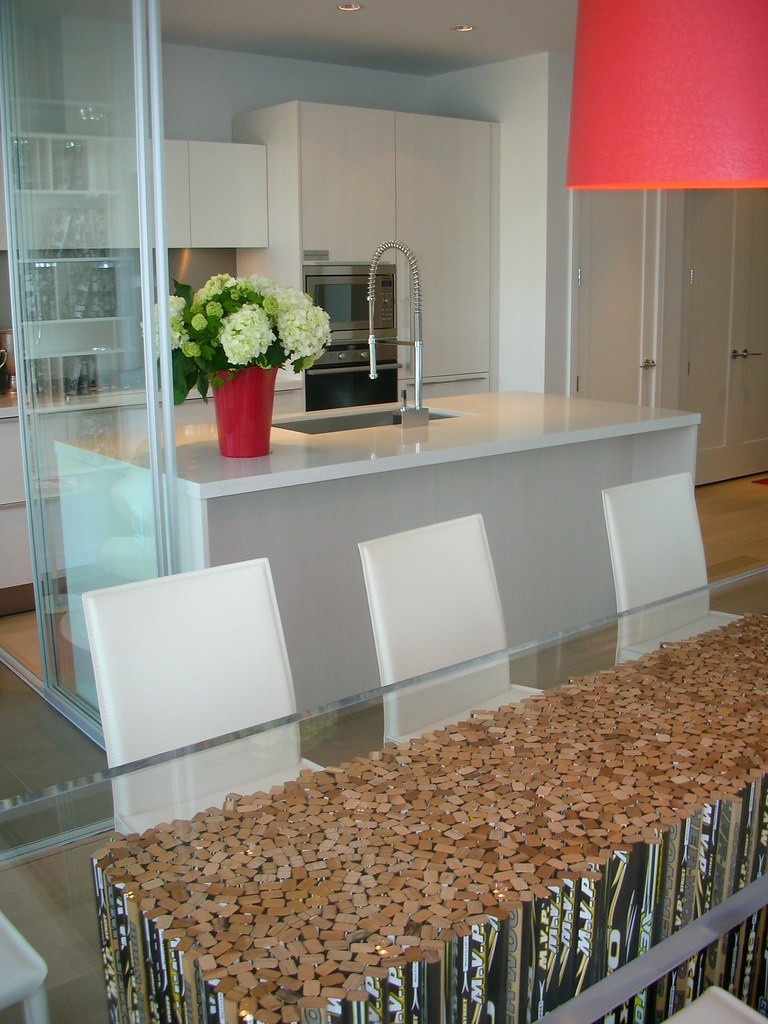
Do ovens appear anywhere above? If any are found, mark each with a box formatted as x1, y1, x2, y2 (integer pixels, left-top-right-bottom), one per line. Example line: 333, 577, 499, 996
300, 344, 402, 412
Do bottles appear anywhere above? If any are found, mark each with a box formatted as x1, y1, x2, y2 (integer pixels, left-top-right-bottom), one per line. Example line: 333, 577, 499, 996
80, 361, 89, 395
73, 272, 116, 323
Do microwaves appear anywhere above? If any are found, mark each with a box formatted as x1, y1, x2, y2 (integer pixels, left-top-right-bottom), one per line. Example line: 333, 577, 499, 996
302, 263, 401, 343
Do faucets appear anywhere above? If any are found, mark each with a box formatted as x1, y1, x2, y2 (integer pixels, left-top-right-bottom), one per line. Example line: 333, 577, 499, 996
366, 239, 432, 427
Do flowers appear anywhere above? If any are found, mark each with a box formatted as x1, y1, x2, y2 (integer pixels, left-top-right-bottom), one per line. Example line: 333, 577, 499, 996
139, 269, 333, 407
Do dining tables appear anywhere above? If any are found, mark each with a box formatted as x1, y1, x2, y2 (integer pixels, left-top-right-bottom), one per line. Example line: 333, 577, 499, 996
1, 563, 767, 1024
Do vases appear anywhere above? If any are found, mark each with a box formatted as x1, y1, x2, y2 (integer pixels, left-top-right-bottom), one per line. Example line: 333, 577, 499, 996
208, 363, 280, 458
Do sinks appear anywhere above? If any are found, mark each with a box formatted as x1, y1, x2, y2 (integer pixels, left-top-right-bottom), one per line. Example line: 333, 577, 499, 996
272, 404, 460, 436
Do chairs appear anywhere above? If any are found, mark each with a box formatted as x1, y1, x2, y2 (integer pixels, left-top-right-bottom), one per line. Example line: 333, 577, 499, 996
79, 555, 327, 834
599, 470, 746, 667
357, 513, 554, 748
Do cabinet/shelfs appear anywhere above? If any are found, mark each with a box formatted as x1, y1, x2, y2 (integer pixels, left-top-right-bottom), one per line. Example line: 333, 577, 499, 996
109, 135, 271, 251
296, 101, 500, 399
1, 132, 119, 421
